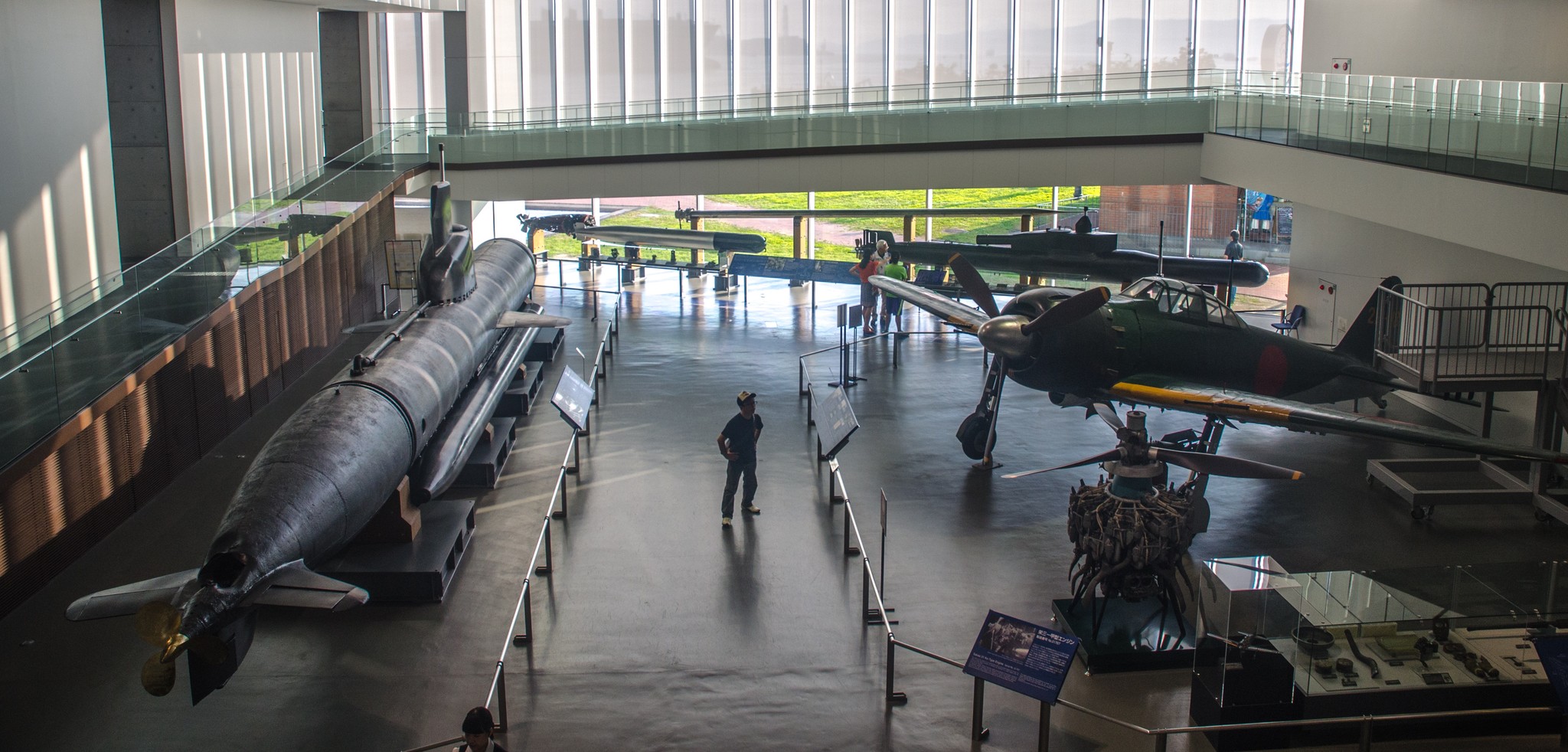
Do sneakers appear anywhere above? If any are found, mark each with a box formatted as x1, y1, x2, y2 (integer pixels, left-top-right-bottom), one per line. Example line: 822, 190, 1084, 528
722, 517, 731, 526
741, 502, 760, 513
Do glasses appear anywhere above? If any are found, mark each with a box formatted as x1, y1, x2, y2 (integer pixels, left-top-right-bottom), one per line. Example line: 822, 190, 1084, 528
743, 401, 757, 407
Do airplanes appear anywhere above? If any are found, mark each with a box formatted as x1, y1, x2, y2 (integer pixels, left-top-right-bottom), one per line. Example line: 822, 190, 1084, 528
65, 126, 578, 701
863, 246, 1408, 480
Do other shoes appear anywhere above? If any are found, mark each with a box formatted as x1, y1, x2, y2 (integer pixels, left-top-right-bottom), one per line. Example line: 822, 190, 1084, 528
863, 325, 875, 335
1230, 302, 1234, 305
880, 329, 909, 336
870, 316, 886, 326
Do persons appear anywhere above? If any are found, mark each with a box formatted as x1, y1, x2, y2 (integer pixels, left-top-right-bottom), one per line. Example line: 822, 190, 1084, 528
881, 250, 910, 337
1215, 229, 1244, 306
715, 391, 763, 527
1250, 192, 1274, 233
869, 238, 892, 325
848, 247, 892, 337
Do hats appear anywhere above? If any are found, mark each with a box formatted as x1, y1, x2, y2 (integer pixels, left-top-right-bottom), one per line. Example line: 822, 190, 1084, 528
736, 391, 756, 409
